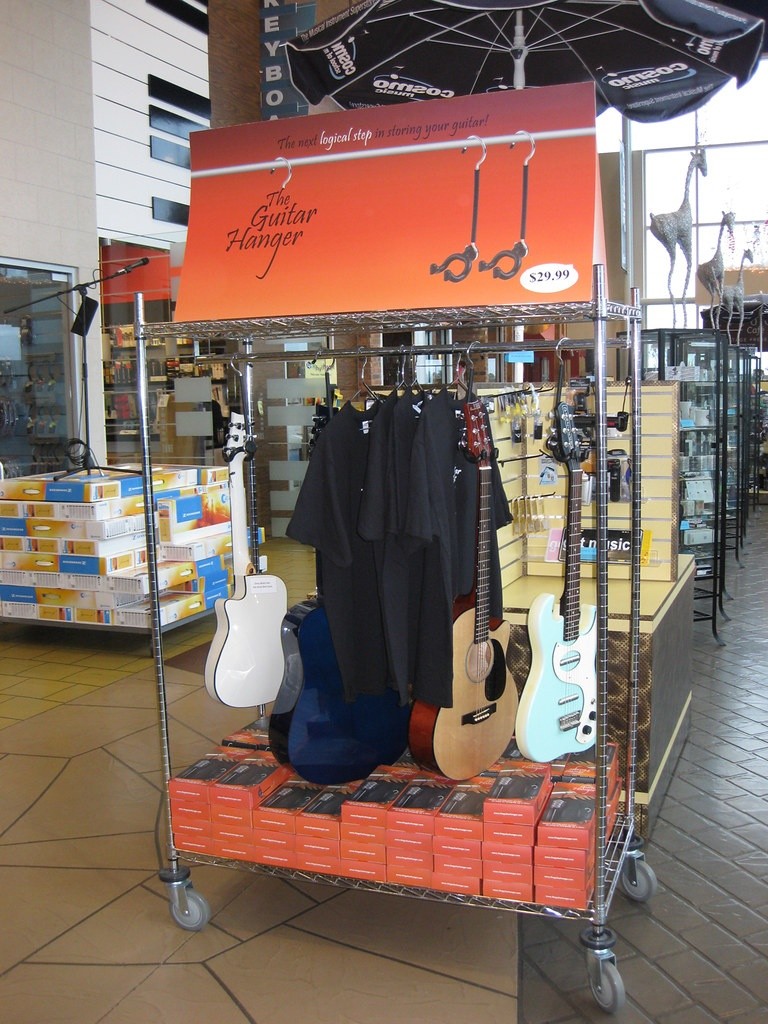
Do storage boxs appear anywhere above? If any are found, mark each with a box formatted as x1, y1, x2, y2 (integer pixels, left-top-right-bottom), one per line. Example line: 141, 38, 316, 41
0, 462, 236, 628
167, 710, 621, 911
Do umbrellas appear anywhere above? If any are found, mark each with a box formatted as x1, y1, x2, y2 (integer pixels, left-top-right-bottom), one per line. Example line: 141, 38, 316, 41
284, 0, 765, 123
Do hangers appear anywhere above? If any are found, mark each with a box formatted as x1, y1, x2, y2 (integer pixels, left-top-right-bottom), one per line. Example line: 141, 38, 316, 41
347, 342, 470, 393
223, 355, 243, 376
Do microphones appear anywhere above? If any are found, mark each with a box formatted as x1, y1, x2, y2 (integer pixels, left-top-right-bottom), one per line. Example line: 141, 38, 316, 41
115, 257, 149, 274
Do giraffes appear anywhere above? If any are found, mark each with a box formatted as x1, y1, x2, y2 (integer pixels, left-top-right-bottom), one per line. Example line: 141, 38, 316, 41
649, 148, 753, 347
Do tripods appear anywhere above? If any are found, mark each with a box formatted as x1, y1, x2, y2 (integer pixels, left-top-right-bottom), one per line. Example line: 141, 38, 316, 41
6, 272, 142, 482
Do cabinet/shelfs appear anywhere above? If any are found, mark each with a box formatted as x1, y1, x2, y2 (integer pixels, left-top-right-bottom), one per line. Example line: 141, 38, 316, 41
615, 328, 768, 645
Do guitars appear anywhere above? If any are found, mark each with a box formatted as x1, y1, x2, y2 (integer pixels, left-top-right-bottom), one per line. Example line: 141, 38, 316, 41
514, 400, 598, 763
203, 411, 290, 709
408, 399, 519, 782
270, 401, 416, 784
